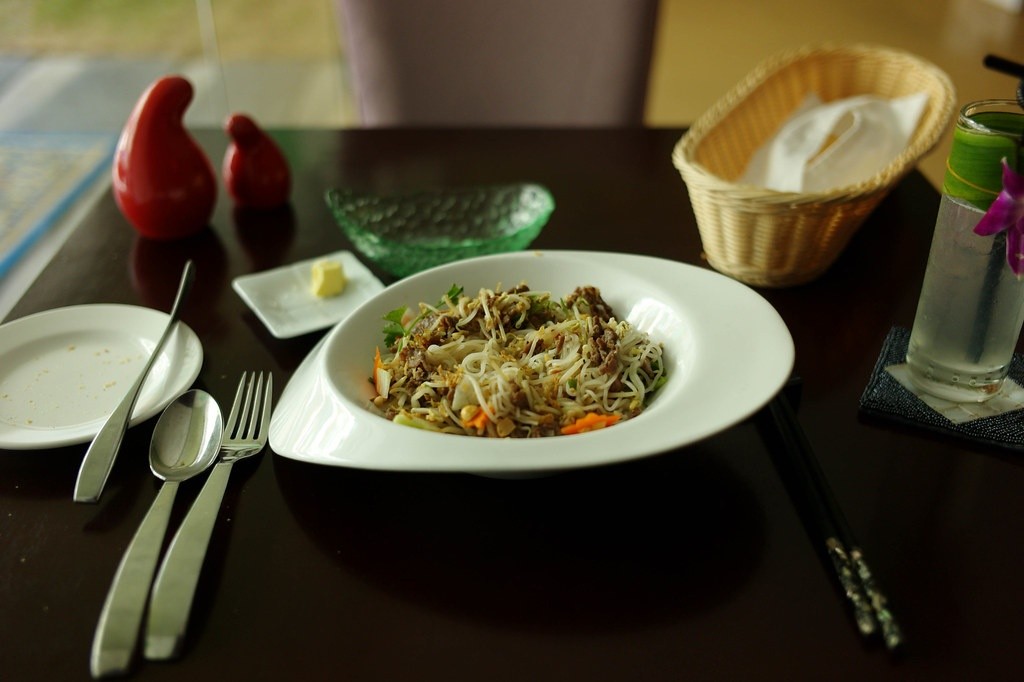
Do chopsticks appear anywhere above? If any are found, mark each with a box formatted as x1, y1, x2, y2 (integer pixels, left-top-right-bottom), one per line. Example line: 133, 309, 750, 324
751, 378, 911, 656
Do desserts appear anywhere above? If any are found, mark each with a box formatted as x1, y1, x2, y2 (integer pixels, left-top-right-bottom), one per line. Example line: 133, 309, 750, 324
311, 259, 346, 298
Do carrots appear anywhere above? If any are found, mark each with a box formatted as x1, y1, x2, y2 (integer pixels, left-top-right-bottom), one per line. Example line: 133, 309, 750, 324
560, 413, 619, 434
460, 406, 496, 429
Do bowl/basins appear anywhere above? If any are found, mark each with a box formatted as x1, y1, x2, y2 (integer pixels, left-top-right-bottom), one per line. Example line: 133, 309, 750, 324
327, 184, 557, 279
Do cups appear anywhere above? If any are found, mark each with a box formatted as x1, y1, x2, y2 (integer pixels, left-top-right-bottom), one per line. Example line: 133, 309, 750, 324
904, 98, 1024, 403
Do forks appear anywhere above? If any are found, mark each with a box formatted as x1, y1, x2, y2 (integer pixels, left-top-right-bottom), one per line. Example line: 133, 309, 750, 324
144, 369, 274, 662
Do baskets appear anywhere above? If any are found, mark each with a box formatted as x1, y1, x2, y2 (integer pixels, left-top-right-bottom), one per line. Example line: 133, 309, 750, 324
672, 38, 956, 289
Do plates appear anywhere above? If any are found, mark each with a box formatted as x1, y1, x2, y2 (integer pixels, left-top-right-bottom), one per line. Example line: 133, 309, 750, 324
267, 246, 798, 473
0, 301, 205, 451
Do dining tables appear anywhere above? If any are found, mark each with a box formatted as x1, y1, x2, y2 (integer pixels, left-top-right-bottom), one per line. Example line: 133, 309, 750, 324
0, 122, 1023, 682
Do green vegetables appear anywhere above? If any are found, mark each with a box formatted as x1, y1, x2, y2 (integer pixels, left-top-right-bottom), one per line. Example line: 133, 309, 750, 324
381, 282, 464, 354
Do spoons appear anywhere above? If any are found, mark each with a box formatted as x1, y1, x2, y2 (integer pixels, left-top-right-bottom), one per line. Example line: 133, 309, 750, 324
89, 389, 225, 680
